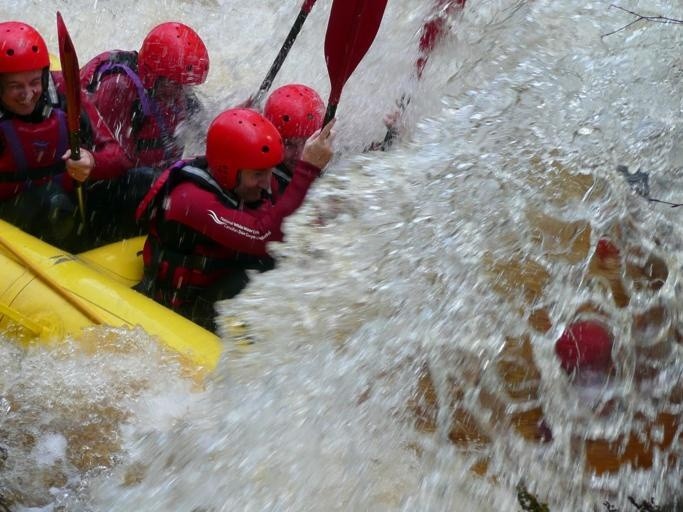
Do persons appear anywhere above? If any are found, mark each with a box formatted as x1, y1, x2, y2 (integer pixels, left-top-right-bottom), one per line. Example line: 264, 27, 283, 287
81, 22, 210, 219
0, 22, 133, 251
143, 110, 338, 333
264, 83, 327, 196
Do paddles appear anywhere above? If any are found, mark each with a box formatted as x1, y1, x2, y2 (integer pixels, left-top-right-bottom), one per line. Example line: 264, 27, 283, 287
56, 10, 86, 236
320, 0, 386, 138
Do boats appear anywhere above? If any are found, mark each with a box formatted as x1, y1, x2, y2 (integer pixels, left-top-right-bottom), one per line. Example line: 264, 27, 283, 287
0, 157, 683, 481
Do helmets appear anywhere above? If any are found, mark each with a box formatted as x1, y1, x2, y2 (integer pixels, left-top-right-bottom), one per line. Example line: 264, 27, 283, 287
205, 108, 286, 189
0, 23, 50, 89
138, 22, 209, 88
265, 84, 326, 140
